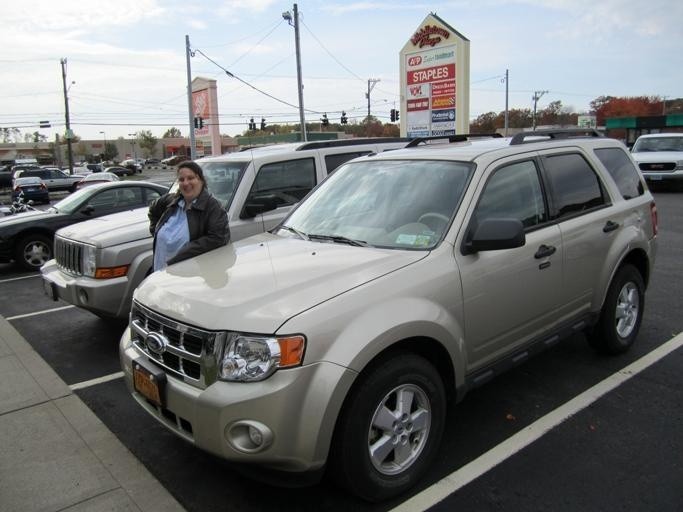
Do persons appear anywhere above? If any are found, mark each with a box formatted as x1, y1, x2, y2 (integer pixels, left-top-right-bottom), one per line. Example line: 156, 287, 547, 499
148, 160, 231, 272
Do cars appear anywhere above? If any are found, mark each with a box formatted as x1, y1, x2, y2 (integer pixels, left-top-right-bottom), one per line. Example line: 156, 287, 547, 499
1, 154, 188, 270
631, 130, 683, 187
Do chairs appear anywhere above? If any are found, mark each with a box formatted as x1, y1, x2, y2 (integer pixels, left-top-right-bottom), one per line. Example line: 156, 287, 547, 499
113, 189, 135, 207
